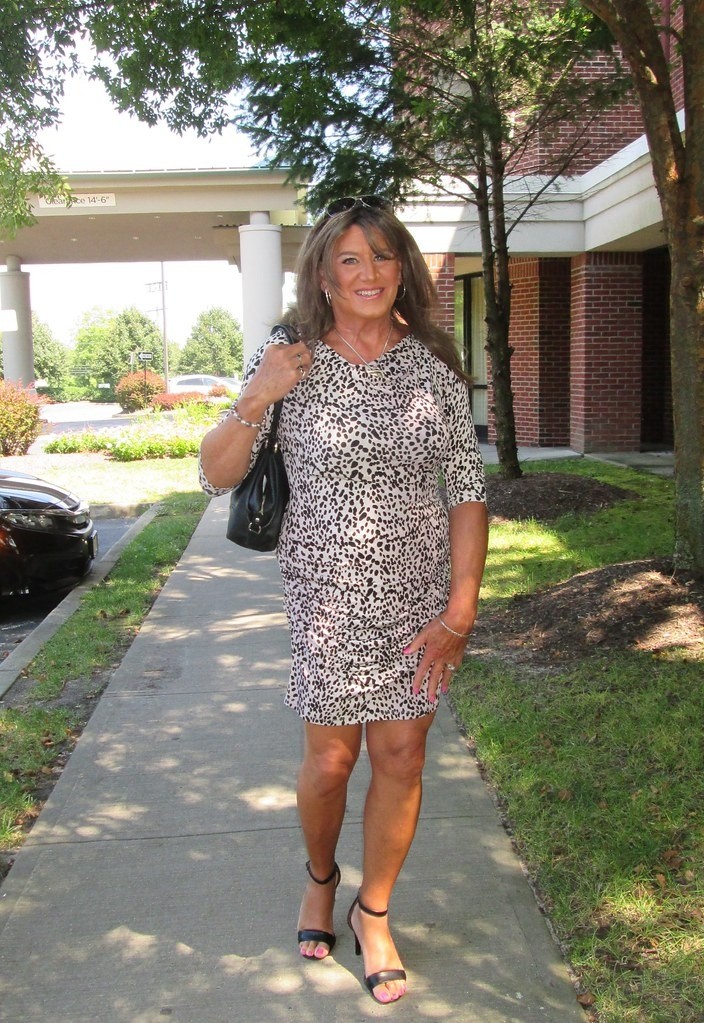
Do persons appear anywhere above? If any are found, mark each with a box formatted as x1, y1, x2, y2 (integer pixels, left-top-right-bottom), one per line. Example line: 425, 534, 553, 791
199, 194, 490, 1003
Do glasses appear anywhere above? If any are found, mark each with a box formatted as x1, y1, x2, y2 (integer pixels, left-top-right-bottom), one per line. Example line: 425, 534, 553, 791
317, 194, 394, 232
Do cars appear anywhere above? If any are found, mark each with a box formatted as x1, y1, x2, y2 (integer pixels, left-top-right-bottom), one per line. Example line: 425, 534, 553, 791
168, 374, 242, 394
0, 468, 98, 619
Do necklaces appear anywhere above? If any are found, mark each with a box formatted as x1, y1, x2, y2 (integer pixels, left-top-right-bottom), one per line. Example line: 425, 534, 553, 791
334, 323, 394, 378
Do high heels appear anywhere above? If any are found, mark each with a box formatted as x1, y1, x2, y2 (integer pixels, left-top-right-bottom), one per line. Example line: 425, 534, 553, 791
348, 889, 409, 1000
298, 857, 341, 960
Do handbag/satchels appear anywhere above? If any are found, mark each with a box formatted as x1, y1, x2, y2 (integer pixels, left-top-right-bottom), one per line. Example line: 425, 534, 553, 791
227, 323, 304, 552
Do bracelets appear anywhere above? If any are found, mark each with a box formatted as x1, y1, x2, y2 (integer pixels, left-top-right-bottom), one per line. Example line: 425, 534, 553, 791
231, 406, 267, 427
438, 617, 472, 638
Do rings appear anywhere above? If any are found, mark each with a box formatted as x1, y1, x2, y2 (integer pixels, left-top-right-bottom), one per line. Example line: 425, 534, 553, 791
296, 354, 302, 361
299, 366, 305, 379
445, 663, 455, 671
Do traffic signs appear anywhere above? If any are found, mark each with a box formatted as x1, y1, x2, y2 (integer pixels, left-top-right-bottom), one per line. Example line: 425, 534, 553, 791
138, 352, 153, 361
